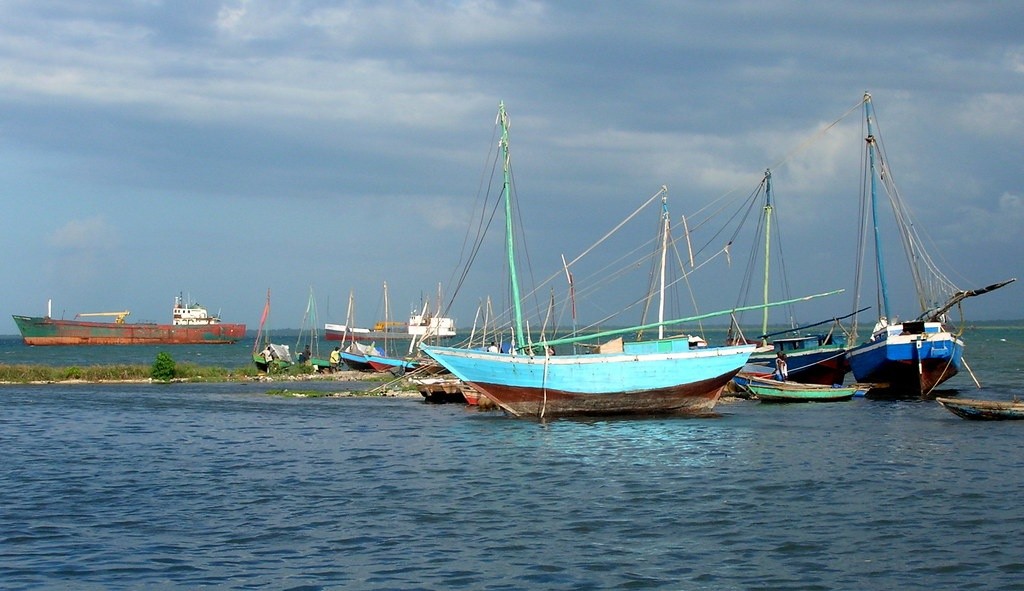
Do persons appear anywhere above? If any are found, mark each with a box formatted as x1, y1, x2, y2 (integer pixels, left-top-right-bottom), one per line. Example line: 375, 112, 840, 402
774, 351, 788, 382
303, 345, 310, 359
262, 347, 273, 363
330, 347, 342, 374
488, 342, 498, 353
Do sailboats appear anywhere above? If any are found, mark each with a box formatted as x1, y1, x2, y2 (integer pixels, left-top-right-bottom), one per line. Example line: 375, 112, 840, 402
724, 169, 861, 391
338, 289, 383, 370
251, 287, 295, 373
362, 280, 421, 373
843, 89, 1016, 397
417, 100, 757, 420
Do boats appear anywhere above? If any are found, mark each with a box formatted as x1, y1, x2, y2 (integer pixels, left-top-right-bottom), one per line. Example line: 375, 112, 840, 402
733, 374, 874, 396
934, 397, 1024, 420
324, 281, 456, 341
294, 284, 344, 372
416, 378, 465, 400
414, 356, 452, 375
460, 384, 497, 405
11, 289, 247, 345
746, 383, 858, 401
